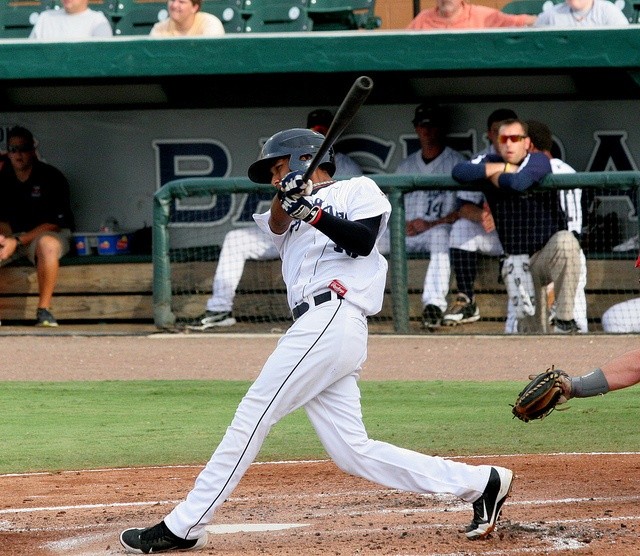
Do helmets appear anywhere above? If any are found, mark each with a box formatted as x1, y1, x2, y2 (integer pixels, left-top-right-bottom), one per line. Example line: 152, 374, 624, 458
248, 127, 336, 183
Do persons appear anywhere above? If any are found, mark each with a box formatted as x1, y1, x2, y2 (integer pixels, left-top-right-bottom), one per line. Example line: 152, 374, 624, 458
148, 0, 228, 36
184, 108, 363, 331
119, 126, 515, 556
406, 0, 540, 29
451, 118, 584, 333
27, 0, 114, 38
532, 0, 631, 28
375, 102, 468, 330
442, 106, 523, 324
1, 122, 77, 329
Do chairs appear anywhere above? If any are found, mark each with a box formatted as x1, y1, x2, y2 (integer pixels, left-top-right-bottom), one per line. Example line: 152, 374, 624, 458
0, 0, 382, 38
502, 0, 639, 26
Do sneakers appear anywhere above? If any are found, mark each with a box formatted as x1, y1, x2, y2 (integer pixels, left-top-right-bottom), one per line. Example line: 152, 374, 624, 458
119, 520, 208, 554
35, 307, 59, 327
186, 310, 236, 329
551, 316, 579, 334
421, 304, 444, 329
442, 291, 481, 327
464, 465, 516, 540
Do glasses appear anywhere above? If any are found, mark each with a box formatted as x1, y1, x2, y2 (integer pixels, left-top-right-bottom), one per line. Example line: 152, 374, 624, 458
6, 143, 33, 153
497, 134, 526, 142
309, 125, 328, 135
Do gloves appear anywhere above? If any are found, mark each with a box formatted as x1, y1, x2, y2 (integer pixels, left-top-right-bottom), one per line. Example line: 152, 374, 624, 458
280, 193, 320, 223
277, 169, 313, 201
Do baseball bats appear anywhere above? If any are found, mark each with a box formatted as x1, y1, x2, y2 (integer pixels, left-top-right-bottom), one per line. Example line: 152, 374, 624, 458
301, 75, 374, 184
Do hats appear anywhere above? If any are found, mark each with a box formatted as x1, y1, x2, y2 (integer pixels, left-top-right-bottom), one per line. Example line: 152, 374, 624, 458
306, 108, 335, 128
411, 99, 449, 126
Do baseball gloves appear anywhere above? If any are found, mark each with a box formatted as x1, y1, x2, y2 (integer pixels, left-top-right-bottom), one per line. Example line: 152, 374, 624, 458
508, 364, 573, 423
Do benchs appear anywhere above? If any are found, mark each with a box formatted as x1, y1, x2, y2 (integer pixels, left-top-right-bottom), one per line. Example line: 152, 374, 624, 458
1, 246, 640, 321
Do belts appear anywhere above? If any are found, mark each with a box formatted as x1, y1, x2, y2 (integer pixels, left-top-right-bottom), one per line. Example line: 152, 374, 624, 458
290, 290, 344, 321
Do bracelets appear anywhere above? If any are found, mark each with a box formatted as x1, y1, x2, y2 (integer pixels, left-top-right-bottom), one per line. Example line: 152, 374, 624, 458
504, 162, 510, 175
